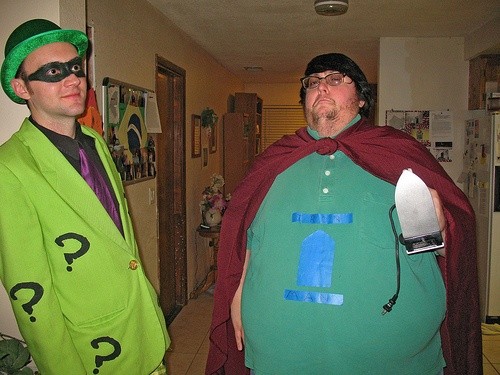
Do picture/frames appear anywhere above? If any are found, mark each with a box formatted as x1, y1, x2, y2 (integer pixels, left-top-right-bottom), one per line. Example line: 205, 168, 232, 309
208, 125, 217, 153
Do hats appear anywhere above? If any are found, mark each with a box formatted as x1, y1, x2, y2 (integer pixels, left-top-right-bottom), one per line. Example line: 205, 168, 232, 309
0, 18, 89, 104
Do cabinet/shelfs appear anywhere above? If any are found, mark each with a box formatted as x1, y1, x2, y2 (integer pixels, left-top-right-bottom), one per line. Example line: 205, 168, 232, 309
469, 54, 500, 111
222, 93, 262, 196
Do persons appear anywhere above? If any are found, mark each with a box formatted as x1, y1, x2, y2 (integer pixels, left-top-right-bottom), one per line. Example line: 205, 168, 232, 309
204, 53, 483, 375
0, 19, 171, 375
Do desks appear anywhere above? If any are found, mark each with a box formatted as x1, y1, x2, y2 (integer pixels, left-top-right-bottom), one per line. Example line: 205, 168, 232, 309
189, 227, 223, 300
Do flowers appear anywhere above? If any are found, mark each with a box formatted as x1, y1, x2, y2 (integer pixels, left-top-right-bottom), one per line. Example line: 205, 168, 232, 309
201, 174, 230, 210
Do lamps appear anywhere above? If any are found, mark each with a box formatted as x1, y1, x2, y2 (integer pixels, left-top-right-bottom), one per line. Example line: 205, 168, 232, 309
314, 0, 349, 16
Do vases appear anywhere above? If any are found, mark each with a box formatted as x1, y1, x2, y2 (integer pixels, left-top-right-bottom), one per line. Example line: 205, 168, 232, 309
202, 206, 210, 225
206, 208, 221, 226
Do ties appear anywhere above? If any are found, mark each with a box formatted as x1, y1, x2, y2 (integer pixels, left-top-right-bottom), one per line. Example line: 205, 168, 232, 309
76, 140, 123, 235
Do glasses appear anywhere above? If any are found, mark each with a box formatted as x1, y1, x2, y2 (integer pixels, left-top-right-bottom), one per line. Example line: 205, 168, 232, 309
300, 71, 347, 89
